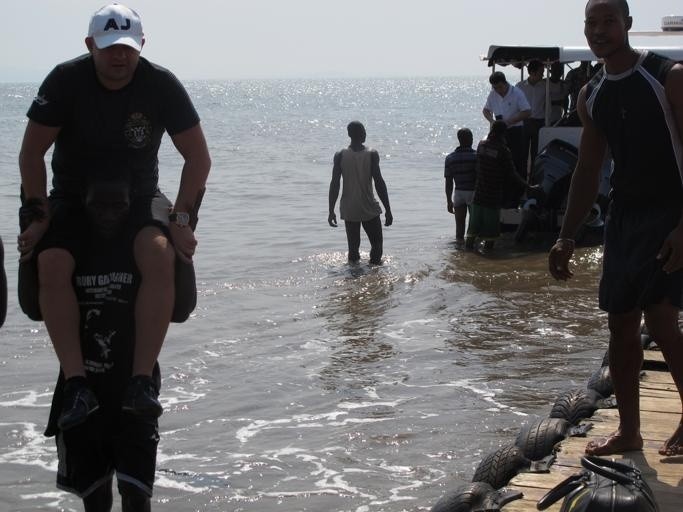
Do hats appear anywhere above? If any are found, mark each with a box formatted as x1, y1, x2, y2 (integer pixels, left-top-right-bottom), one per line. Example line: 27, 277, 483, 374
88, 3, 143, 53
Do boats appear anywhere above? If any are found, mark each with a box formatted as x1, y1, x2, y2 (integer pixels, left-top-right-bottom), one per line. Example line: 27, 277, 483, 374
481, 39, 682, 262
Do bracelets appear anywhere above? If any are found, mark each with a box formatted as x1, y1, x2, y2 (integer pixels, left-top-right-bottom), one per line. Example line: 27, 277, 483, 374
556, 238, 575, 243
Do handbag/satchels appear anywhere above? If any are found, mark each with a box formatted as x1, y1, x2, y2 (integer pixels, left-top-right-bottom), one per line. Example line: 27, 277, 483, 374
561, 457, 659, 511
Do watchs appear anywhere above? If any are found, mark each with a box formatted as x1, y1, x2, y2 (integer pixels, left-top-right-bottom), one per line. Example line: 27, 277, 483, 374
167, 212, 192, 226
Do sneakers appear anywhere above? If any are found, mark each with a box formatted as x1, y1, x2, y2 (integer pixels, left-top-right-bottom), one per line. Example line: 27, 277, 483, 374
58, 379, 100, 431
121, 378, 163, 420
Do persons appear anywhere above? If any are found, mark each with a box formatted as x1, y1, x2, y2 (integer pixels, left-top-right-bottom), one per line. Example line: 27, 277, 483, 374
17, 4, 210, 429
464, 121, 542, 252
548, 0, 683, 457
329, 121, 393, 265
443, 127, 477, 240
514, 60, 602, 176
17, 175, 212, 511
482, 71, 533, 174
